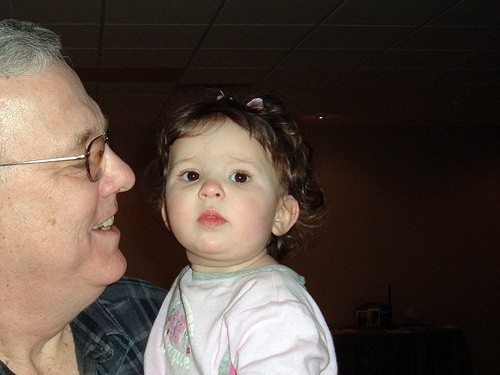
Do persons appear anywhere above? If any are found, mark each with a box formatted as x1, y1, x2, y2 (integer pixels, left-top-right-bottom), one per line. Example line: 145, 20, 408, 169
1, 19, 172, 375
141, 92, 338, 375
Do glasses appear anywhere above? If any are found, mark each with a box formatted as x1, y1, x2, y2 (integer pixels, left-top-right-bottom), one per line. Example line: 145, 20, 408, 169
0, 130, 112, 183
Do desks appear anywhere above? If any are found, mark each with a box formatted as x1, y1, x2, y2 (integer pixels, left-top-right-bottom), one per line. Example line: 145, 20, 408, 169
328, 322, 472, 375
